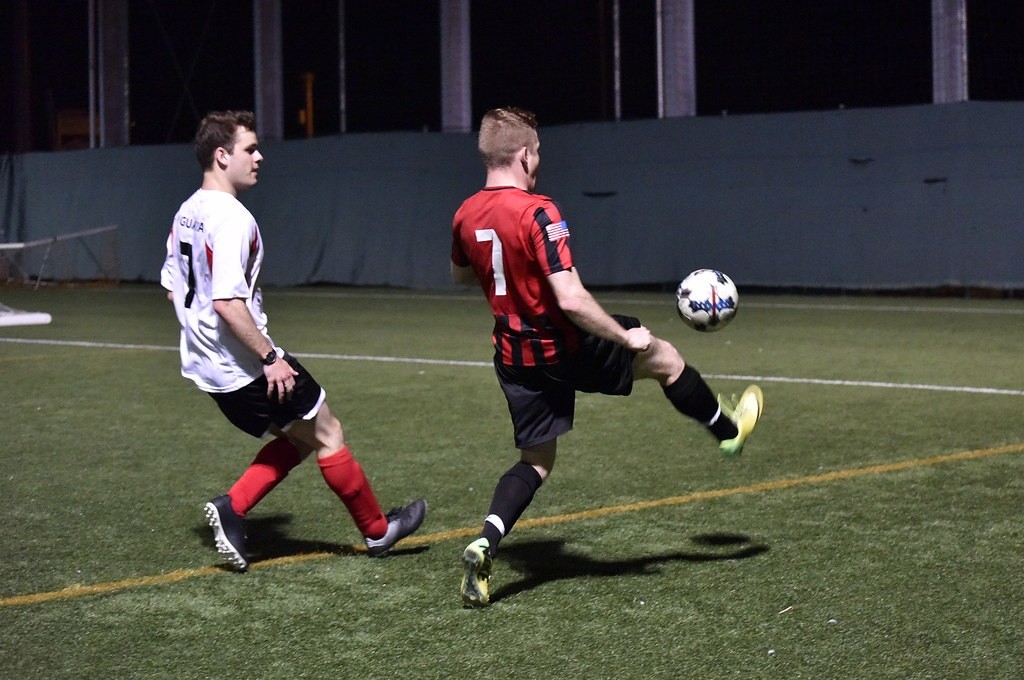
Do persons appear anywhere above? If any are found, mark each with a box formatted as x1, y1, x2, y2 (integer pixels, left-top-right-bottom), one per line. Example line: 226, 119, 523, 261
449, 110, 764, 608
161, 111, 429, 570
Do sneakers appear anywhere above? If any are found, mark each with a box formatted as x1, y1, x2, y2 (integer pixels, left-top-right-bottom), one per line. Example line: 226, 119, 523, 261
204, 495, 249, 573
459, 537, 492, 607
364, 499, 427, 558
717, 385, 764, 455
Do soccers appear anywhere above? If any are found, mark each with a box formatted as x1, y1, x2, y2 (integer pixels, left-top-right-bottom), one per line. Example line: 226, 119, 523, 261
677, 268, 740, 334
